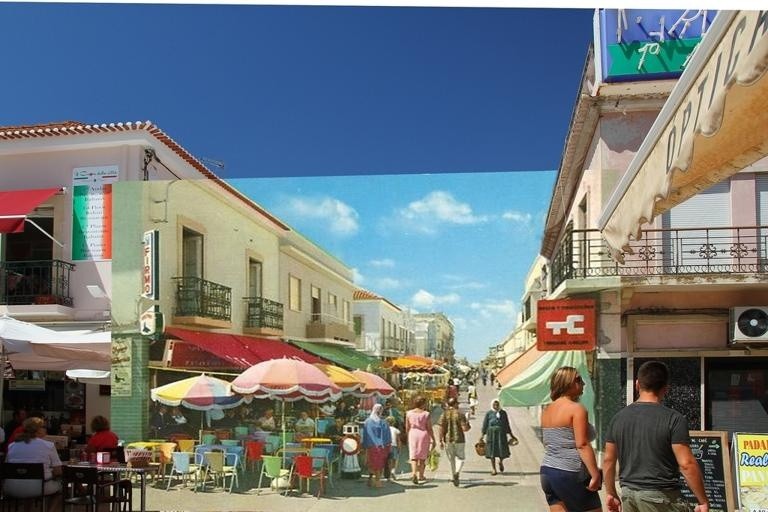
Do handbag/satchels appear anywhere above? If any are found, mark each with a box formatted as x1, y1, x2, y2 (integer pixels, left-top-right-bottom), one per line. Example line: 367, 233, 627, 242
474, 438, 487, 456
469, 388, 477, 399
577, 460, 603, 491
426, 446, 441, 473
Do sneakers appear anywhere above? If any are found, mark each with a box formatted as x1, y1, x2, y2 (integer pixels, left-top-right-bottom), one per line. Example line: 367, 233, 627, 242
491, 471, 497, 475
498, 462, 505, 472
450, 472, 460, 487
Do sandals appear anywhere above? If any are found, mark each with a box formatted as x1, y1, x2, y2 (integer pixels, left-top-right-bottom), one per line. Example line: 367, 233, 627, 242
419, 477, 427, 481
412, 474, 419, 485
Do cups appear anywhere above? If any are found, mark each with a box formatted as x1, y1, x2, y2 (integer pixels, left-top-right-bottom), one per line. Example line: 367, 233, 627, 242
69, 448, 96, 465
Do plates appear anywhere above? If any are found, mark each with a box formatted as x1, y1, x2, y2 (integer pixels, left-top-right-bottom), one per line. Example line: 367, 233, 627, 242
65, 396, 83, 406
66, 381, 82, 392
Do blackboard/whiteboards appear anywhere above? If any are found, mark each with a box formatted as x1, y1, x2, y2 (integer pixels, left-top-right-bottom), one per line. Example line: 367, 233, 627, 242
679, 430, 735, 512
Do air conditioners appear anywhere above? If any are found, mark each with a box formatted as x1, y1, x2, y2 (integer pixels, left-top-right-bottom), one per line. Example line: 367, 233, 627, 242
728, 304, 767, 343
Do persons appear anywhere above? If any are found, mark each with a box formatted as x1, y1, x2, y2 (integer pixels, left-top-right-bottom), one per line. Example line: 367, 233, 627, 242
405, 397, 436, 483
604, 361, 711, 512
386, 416, 401, 481
539, 366, 604, 512
149, 397, 400, 444
426, 367, 495, 414
363, 403, 391, 487
480, 399, 516, 476
439, 397, 471, 486
0, 406, 119, 512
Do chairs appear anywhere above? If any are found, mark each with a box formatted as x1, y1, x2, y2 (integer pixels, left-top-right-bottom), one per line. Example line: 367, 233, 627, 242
0, 433, 159, 510
122, 413, 357, 498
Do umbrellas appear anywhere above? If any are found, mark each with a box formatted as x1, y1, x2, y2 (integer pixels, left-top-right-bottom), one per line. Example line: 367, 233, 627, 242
230, 354, 341, 429
378, 355, 449, 380
0, 312, 111, 385
349, 369, 395, 400
150, 373, 252, 431
312, 362, 367, 395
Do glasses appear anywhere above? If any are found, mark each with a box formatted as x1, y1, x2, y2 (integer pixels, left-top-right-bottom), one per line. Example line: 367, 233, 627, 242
574, 376, 583, 385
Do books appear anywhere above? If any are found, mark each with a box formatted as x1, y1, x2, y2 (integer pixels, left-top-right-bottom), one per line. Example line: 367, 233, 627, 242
580, 463, 602, 490
